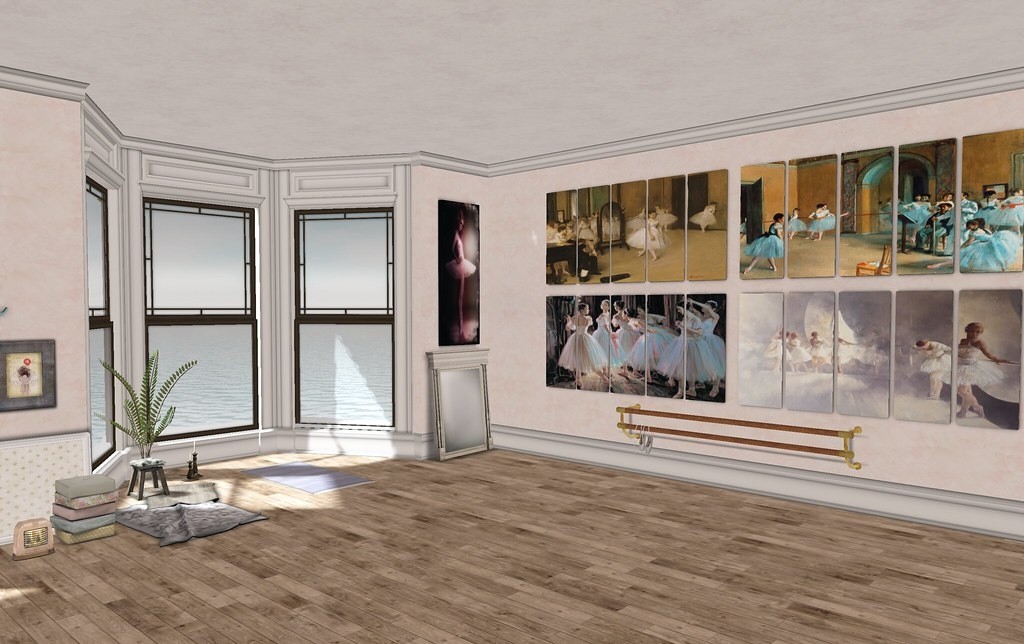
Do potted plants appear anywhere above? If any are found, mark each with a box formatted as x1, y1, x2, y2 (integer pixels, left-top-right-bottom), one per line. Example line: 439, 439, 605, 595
93, 349, 198, 479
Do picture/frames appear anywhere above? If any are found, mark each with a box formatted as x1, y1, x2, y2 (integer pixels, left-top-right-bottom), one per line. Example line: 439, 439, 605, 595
740, 128, 1024, 279
545, 293, 727, 403
545, 170, 728, 285
737, 286, 1021, 432
0, 339, 58, 410
437, 199, 483, 346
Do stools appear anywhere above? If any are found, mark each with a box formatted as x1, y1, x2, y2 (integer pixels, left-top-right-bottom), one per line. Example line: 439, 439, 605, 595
126, 459, 170, 501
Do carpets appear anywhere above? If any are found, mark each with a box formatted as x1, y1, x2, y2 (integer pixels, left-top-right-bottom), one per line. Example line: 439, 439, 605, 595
115, 498, 269, 547
139, 482, 220, 510
